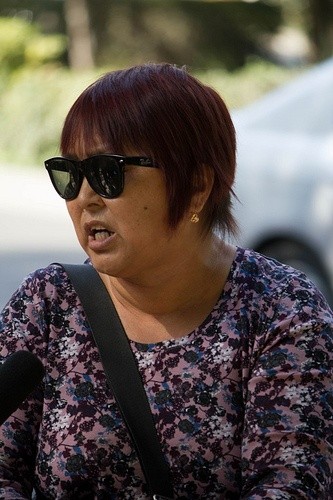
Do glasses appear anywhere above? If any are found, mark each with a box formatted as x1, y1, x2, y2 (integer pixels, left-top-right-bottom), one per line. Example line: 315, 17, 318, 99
43, 153, 167, 201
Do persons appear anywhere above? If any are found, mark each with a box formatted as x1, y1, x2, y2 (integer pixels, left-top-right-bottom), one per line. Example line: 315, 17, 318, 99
0, 64, 333, 500
